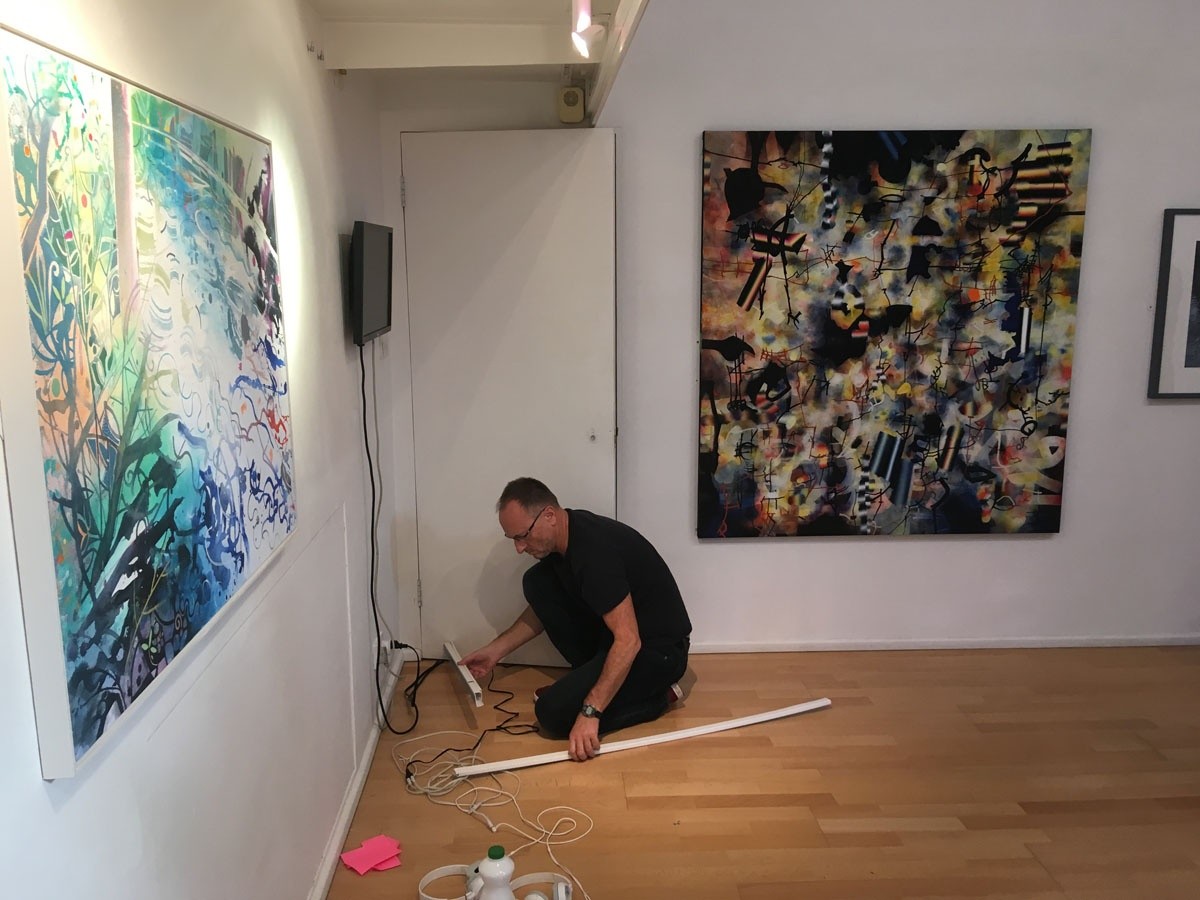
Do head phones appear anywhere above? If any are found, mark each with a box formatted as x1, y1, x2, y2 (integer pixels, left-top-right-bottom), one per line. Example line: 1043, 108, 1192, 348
508, 871, 575, 899
418, 858, 485, 900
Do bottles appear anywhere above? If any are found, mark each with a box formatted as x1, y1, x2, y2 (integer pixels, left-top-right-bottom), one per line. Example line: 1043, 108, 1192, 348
476, 844, 517, 900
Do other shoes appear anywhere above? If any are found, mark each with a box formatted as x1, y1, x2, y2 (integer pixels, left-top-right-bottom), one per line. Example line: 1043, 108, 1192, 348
665, 682, 683, 704
533, 686, 551, 702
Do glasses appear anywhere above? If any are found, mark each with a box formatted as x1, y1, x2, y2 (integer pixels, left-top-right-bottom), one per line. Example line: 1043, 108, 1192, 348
505, 507, 546, 542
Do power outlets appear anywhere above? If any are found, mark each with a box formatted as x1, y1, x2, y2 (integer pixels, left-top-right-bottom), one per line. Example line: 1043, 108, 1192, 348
380, 639, 394, 664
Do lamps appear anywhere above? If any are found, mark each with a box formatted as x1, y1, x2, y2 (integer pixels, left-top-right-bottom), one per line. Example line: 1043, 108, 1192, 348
570, 17, 610, 58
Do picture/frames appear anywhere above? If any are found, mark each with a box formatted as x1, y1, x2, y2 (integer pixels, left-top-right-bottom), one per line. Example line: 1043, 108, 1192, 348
0, 22, 301, 784
1146, 208, 1200, 400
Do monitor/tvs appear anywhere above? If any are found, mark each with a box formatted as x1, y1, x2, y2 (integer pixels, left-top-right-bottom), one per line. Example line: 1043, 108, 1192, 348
347, 221, 394, 347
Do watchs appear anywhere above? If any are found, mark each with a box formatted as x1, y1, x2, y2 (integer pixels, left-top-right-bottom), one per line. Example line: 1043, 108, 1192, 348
580, 703, 603, 719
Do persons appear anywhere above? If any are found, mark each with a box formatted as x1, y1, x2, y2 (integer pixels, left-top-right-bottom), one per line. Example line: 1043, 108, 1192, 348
456, 477, 693, 761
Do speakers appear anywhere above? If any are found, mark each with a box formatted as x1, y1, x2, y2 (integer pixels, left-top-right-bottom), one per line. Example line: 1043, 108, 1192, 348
559, 86, 585, 123
